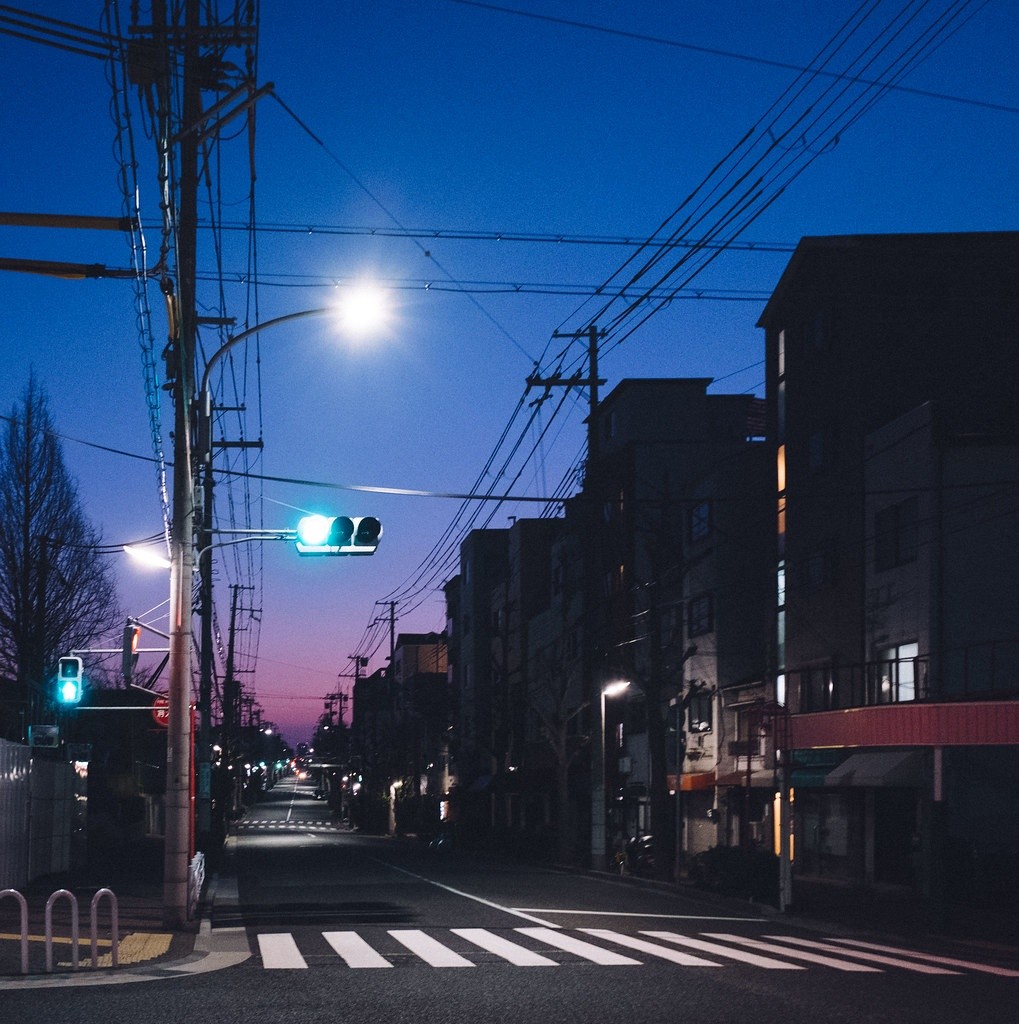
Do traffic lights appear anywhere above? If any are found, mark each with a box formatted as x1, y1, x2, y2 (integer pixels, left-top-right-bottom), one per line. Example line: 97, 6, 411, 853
57, 656, 83, 706
294, 513, 386, 556
123, 626, 140, 679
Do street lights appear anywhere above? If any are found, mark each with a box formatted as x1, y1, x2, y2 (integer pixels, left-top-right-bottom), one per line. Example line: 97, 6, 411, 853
161, 282, 395, 930
